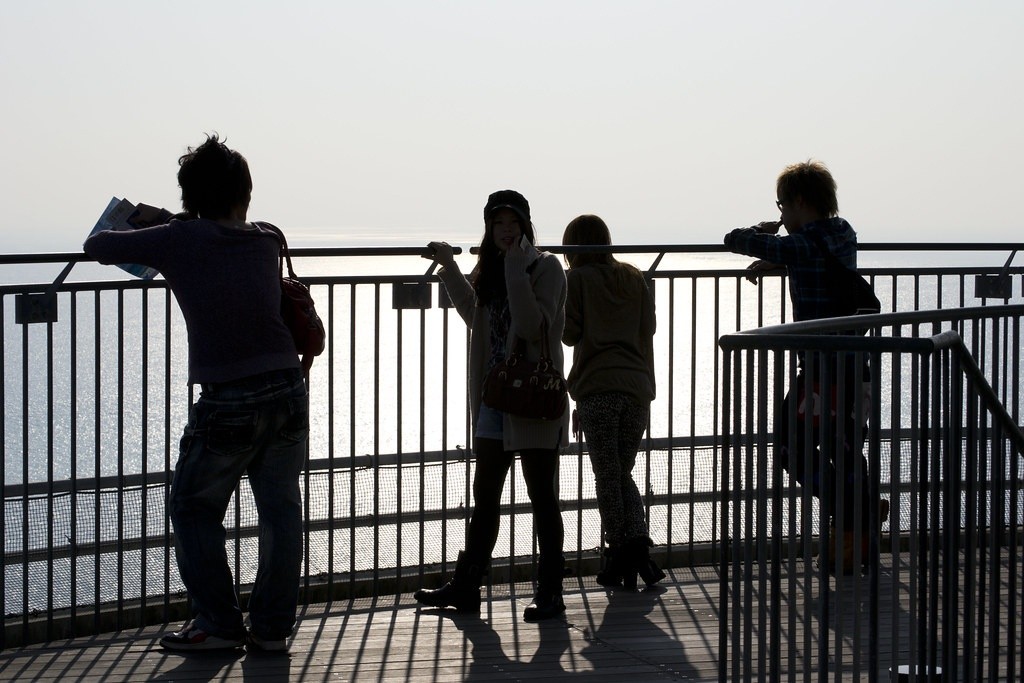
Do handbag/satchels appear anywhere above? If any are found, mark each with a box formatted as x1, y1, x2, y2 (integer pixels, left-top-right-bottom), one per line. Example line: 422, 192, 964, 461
255, 221, 326, 380
481, 304, 568, 422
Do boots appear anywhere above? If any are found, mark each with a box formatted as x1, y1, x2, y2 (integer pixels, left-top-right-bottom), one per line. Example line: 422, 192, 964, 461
414, 550, 490, 611
523, 555, 566, 621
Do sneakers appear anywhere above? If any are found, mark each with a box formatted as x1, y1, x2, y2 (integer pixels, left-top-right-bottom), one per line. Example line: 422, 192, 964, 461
159, 613, 287, 651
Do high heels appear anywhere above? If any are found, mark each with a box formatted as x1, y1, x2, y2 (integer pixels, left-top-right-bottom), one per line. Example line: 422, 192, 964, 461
595, 536, 666, 590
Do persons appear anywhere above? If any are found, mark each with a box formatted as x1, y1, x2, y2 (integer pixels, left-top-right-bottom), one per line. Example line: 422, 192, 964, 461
83, 130, 310, 652
561, 215, 667, 589
414, 189, 571, 624
725, 159, 889, 575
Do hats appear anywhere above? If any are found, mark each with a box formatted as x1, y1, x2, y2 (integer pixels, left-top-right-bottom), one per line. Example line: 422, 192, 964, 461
484, 190, 530, 225
